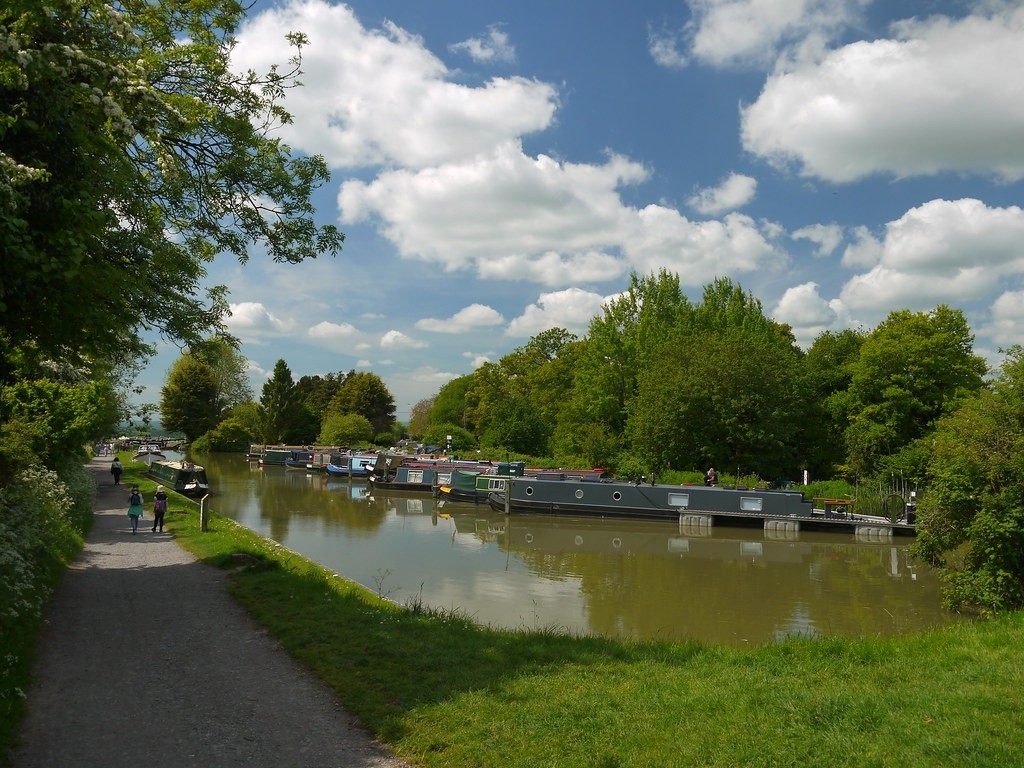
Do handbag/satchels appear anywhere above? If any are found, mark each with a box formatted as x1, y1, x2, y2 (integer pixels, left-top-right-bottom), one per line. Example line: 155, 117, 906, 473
120, 470, 123, 474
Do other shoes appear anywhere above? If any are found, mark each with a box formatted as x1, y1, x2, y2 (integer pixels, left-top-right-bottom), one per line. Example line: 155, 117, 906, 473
133, 530, 137, 534
151, 528, 156, 532
159, 529, 163, 534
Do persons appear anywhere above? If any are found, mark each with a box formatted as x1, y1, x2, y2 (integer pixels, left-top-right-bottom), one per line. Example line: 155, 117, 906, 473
703, 468, 719, 487
95, 440, 133, 457
152, 485, 168, 533
127, 485, 144, 535
110, 457, 123, 486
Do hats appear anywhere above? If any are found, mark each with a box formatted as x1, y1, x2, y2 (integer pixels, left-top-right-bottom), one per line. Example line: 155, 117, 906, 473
157, 485, 163, 493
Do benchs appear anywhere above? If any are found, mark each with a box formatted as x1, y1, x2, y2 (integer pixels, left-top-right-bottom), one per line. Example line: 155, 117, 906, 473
812, 497, 856, 520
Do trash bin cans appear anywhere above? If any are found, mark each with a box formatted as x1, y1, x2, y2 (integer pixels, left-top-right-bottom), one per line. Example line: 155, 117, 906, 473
498, 461, 525, 475
905, 495, 917, 525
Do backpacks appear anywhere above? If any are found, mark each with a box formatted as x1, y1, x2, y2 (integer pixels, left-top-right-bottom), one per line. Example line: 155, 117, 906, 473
131, 492, 141, 506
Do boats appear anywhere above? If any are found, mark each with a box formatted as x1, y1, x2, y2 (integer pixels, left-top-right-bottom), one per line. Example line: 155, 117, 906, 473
137, 444, 162, 455
245, 440, 608, 506
146, 458, 209, 497
